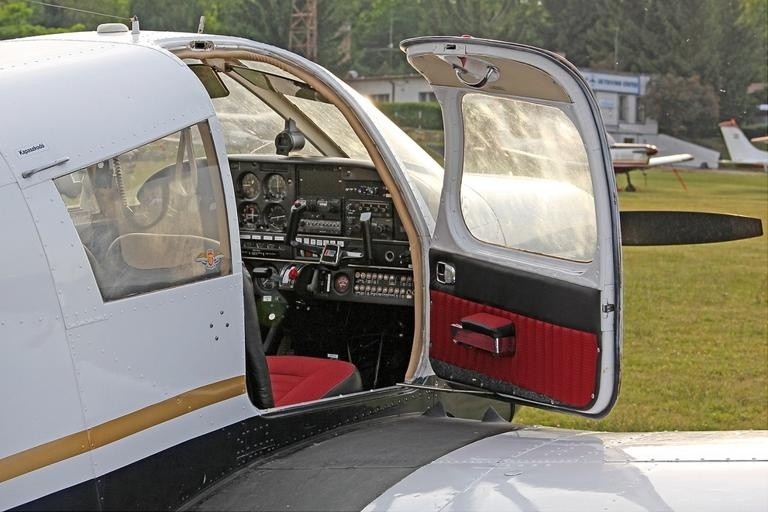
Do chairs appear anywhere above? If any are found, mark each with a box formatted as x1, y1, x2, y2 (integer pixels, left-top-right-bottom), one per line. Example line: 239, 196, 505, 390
106, 234, 363, 408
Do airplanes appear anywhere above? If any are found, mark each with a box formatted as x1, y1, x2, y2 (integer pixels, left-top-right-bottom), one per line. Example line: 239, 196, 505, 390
602, 124, 696, 192
713, 117, 768, 176
0, 11, 767, 512
